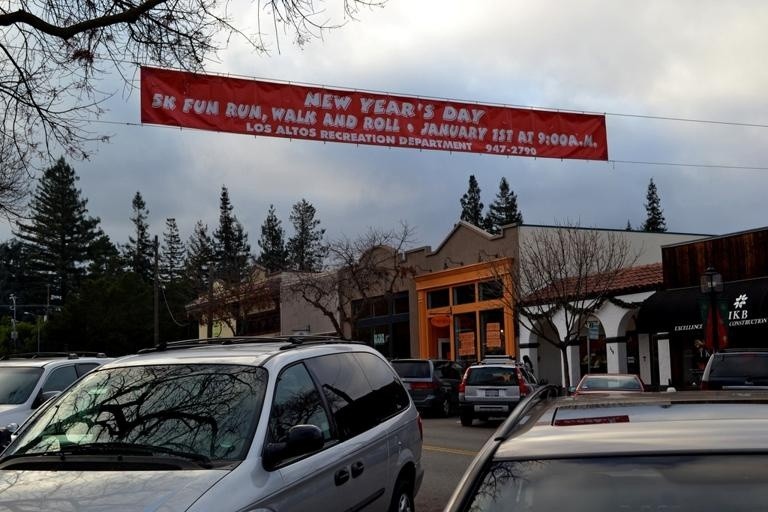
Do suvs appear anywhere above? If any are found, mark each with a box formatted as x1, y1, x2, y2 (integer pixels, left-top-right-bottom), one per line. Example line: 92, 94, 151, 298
0, 348, 121, 459
0, 328, 430, 511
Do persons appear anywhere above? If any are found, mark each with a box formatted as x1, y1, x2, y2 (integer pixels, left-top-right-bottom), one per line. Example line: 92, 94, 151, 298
522, 356, 534, 374
695, 339, 715, 370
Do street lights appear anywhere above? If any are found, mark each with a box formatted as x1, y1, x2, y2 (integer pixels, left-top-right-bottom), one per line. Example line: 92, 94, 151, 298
698, 266, 726, 353
23, 311, 42, 352
153, 283, 167, 345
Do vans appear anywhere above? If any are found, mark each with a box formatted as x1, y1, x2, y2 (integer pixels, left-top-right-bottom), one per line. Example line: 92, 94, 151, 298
389, 353, 468, 418
